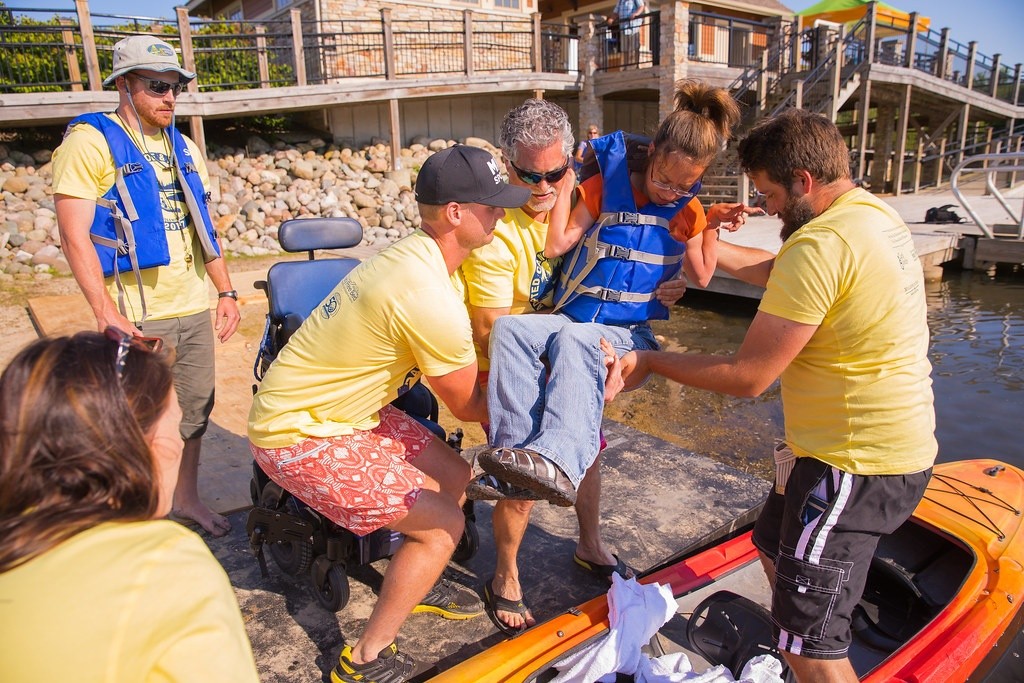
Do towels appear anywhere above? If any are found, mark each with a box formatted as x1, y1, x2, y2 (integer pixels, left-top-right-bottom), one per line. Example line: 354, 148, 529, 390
548, 568, 787, 683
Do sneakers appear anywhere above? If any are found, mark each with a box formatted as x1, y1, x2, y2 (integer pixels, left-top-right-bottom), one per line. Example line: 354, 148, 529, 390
409, 571, 492, 619
330, 636, 440, 683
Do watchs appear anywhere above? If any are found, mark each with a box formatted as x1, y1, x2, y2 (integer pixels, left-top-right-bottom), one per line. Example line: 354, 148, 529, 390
219, 290, 238, 301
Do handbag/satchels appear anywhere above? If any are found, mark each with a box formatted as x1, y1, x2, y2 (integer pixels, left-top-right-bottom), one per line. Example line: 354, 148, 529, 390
925, 203, 968, 222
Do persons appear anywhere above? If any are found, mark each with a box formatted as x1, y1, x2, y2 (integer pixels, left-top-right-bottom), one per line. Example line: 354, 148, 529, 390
244, 143, 616, 683
620, 106, 938, 683
0, 323, 262, 683
574, 125, 600, 180
51, 35, 241, 538
460, 97, 686, 637
607, 0, 646, 73
464, 76, 766, 508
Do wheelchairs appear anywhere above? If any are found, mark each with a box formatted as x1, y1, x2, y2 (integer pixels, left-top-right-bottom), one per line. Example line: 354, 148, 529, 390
245, 217, 482, 614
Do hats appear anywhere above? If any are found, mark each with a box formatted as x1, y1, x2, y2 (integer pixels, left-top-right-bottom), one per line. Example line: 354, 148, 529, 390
415, 142, 532, 208
102, 35, 197, 87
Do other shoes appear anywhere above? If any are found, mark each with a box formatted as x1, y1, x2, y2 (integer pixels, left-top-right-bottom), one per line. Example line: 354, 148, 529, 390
636, 64, 639, 69
622, 66, 626, 71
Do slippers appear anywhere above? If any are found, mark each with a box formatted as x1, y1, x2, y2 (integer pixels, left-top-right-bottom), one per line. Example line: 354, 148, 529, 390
574, 550, 642, 587
484, 578, 537, 637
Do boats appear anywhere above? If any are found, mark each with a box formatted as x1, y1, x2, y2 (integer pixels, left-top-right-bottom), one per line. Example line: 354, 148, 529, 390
422, 454, 1024, 683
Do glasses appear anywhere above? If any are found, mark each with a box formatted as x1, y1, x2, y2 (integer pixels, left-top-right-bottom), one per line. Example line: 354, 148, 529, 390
589, 132, 597, 134
129, 71, 185, 97
650, 155, 704, 196
104, 325, 145, 380
511, 154, 569, 187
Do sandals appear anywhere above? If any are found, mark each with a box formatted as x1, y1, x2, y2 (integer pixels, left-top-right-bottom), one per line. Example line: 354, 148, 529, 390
478, 447, 577, 507
465, 472, 543, 501
